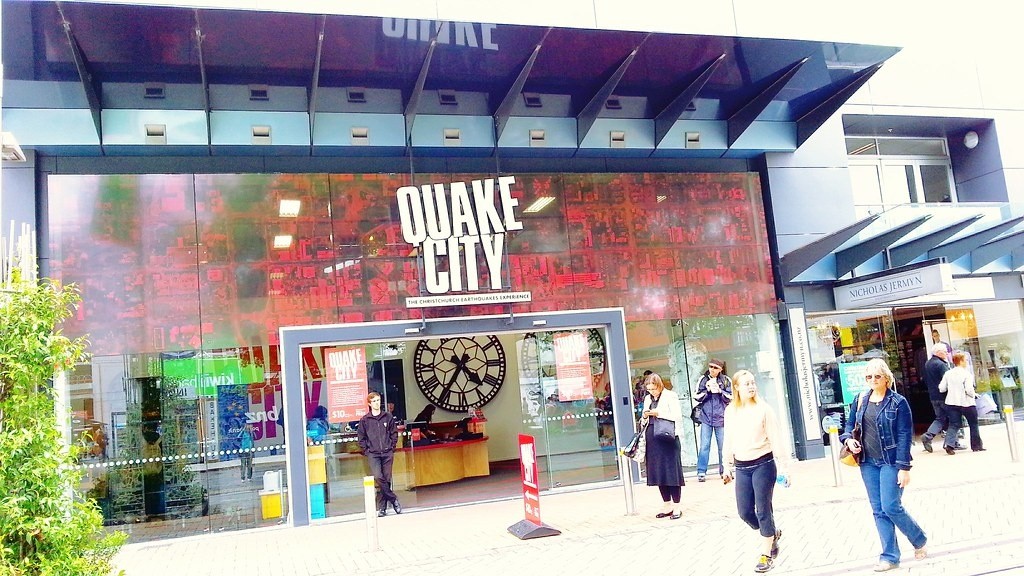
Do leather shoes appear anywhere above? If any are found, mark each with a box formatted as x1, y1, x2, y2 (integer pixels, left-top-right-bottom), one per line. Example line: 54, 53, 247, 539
378, 496, 401, 517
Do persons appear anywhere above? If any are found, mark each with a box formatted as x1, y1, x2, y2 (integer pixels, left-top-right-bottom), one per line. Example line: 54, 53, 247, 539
838, 358, 928, 572
414, 404, 436, 422
357, 392, 401, 517
921, 342, 987, 455
307, 405, 330, 503
722, 371, 792, 573
142, 417, 164, 512
932, 330, 953, 363
387, 400, 401, 423
642, 373, 685, 520
692, 358, 735, 482
238, 423, 255, 483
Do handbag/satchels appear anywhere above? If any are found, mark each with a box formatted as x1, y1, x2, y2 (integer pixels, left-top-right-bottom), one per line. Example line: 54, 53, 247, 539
840, 391, 867, 467
690, 403, 702, 427
619, 416, 649, 464
654, 417, 676, 441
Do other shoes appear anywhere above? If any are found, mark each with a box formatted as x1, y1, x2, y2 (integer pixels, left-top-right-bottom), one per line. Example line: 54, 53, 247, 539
954, 445, 966, 450
921, 433, 933, 453
698, 472, 723, 481
656, 509, 682, 520
944, 444, 954, 455
973, 447, 986, 452
242, 479, 252, 483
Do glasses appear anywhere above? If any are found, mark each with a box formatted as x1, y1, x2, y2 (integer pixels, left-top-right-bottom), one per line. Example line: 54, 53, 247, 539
709, 365, 719, 369
646, 386, 659, 391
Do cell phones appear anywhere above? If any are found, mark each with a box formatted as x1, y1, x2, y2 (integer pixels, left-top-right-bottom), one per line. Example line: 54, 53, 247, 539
853, 442, 859, 448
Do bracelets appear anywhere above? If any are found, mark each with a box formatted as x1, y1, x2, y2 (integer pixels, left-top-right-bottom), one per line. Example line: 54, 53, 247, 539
844, 438, 849, 446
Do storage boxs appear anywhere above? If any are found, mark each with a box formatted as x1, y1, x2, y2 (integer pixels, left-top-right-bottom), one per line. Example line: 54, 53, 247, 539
258, 487, 287, 520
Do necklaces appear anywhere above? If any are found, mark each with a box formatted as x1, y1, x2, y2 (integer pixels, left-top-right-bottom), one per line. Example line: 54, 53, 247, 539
871, 389, 883, 406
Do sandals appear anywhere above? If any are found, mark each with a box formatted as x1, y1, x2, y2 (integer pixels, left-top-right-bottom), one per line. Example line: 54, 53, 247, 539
873, 543, 928, 573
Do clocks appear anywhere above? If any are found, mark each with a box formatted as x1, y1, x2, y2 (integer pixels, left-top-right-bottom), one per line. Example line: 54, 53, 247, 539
414, 334, 506, 412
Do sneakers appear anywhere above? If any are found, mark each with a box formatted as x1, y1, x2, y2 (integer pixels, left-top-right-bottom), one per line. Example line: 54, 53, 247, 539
754, 528, 782, 572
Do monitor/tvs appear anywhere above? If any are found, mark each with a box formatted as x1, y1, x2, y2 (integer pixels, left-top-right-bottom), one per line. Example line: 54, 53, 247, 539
454, 417, 472, 428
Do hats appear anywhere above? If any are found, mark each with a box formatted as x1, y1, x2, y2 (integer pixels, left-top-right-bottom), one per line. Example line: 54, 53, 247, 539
709, 358, 724, 370
